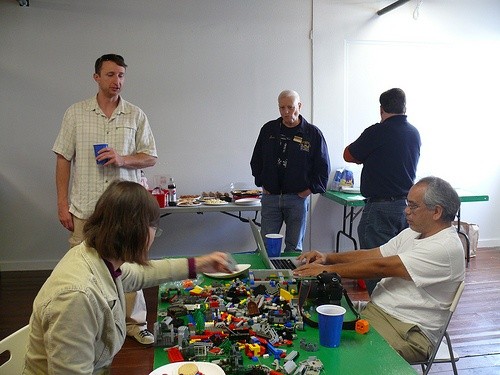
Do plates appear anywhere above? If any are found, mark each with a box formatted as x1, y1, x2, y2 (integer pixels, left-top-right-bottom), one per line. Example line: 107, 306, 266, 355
343, 188, 360, 194
204, 264, 251, 278
178, 202, 201, 206
204, 202, 228, 205
235, 198, 261, 205
149, 362, 226, 375
201, 200, 222, 202
178, 196, 200, 200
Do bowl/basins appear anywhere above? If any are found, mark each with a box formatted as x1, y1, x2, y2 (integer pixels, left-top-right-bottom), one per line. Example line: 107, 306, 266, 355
231, 190, 263, 199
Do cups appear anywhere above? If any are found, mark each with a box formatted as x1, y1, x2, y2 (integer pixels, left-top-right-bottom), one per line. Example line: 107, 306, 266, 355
331, 170, 354, 190
317, 305, 346, 348
265, 234, 283, 258
93, 144, 108, 165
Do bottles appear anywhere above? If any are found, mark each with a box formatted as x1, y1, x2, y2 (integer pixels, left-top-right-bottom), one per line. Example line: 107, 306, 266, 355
168, 177, 176, 206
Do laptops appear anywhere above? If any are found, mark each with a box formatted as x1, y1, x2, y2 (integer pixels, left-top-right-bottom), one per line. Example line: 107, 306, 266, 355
248, 216, 318, 279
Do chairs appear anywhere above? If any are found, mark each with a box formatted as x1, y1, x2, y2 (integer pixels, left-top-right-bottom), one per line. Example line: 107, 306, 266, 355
408, 231, 471, 375
0, 323, 30, 375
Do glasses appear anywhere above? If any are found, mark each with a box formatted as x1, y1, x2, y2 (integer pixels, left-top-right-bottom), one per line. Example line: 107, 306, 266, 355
404, 198, 432, 210
146, 227, 162, 237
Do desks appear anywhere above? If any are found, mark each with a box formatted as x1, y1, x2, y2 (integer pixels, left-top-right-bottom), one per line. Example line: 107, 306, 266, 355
160, 198, 263, 227
323, 190, 489, 263
152, 251, 418, 375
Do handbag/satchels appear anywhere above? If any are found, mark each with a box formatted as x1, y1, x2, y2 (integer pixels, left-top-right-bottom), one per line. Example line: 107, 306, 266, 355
453, 220, 479, 257
330, 165, 354, 192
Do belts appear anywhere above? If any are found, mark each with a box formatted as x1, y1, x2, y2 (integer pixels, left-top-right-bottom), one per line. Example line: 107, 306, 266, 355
363, 195, 407, 202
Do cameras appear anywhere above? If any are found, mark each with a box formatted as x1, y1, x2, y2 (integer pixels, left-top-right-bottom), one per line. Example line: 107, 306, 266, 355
298, 270, 343, 310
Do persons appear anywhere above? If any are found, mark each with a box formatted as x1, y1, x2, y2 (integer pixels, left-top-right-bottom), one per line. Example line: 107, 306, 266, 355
250, 89, 331, 253
292, 176, 466, 363
21, 178, 238, 375
343, 87, 421, 297
51, 54, 158, 249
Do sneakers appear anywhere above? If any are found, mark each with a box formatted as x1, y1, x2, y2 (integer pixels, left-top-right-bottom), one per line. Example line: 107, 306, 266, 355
134, 329, 155, 345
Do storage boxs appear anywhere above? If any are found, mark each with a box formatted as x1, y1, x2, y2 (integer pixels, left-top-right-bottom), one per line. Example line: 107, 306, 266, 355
147, 189, 168, 208
